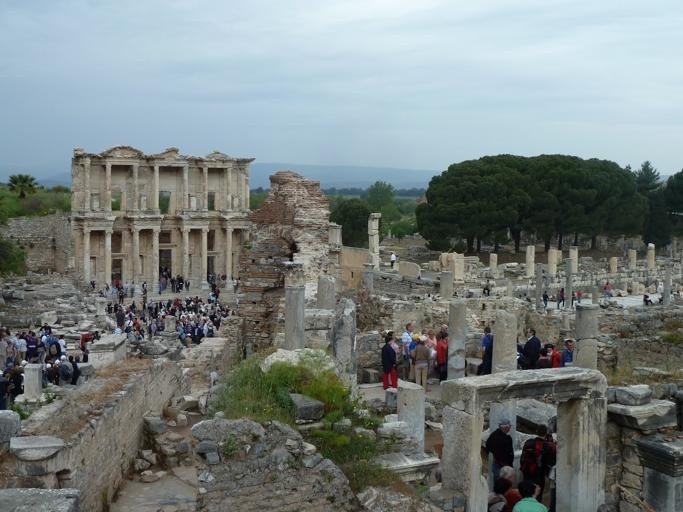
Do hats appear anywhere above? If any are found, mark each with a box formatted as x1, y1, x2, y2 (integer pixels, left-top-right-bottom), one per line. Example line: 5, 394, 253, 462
413, 334, 419, 341
47, 355, 67, 368
420, 335, 428, 341
21, 360, 27, 365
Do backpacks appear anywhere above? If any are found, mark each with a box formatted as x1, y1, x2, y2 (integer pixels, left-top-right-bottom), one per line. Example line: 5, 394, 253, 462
519, 438, 546, 477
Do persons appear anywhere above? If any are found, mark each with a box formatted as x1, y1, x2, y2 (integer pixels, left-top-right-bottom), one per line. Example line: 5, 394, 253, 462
644, 289, 680, 306
518, 287, 582, 309
480, 416, 557, 512
381, 322, 448, 390
482, 280, 491, 296
389, 251, 396, 269
0, 265, 241, 413
479, 303, 488, 327
604, 282, 611, 300
479, 326, 493, 375
516, 327, 576, 370
